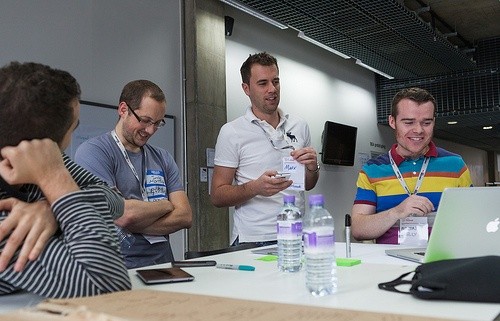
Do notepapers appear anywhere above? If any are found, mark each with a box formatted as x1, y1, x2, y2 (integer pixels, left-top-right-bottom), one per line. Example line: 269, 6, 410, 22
336, 258, 361, 267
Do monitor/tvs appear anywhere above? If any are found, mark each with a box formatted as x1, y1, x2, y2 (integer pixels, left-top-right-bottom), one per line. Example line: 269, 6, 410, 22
321, 121, 357, 166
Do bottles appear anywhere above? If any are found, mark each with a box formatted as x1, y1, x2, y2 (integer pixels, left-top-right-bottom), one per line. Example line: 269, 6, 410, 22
303, 194, 335, 297
277, 195, 303, 273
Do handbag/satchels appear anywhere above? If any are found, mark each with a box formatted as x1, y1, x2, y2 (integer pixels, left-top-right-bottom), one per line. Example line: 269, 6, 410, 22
377, 255, 500, 303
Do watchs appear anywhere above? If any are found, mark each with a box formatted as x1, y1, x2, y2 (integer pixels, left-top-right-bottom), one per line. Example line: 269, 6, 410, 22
307, 161, 320, 173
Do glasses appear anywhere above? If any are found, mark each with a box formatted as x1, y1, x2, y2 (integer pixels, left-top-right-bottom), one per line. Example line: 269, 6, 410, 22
128, 105, 166, 129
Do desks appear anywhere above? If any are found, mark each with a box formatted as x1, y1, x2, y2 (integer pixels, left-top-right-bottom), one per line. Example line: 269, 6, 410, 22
0, 240, 500, 321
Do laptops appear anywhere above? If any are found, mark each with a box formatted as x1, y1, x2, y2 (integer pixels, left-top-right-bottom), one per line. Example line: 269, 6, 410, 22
384, 186, 500, 265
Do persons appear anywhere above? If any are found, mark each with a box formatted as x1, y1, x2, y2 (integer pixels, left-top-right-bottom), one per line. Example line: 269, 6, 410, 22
73, 79, 192, 267
211, 53, 320, 247
351, 88, 474, 244
0, 62, 132, 300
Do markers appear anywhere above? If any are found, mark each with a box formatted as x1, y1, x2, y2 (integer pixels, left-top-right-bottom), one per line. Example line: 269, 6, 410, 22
345, 214, 352, 258
216, 264, 255, 271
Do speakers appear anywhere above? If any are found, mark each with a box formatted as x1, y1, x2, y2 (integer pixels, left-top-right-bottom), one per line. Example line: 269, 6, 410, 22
224, 16, 234, 36
